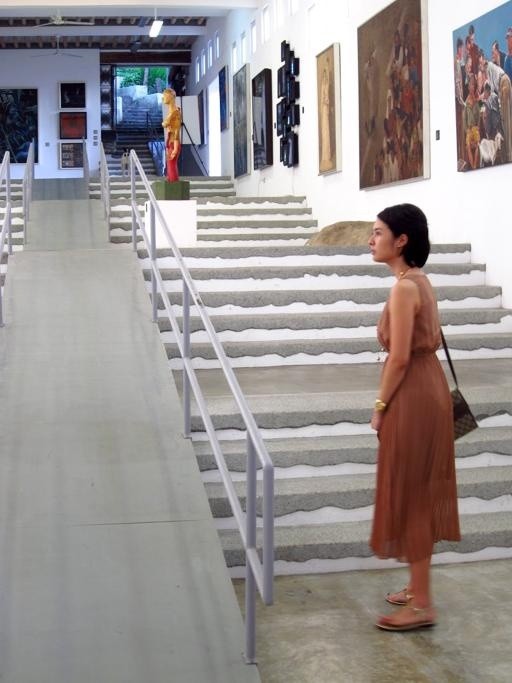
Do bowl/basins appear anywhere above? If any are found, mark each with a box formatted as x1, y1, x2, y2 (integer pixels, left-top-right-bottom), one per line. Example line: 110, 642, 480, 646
395, 265, 412, 281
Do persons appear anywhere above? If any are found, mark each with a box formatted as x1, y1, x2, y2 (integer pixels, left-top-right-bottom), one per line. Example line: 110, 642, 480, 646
362, 15, 512, 185
159, 85, 188, 181
364, 201, 461, 632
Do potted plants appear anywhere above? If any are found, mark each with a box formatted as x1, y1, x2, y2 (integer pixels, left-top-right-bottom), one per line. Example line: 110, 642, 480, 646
59, 140, 87, 169
250, 38, 301, 171
59, 80, 87, 109
58, 110, 89, 140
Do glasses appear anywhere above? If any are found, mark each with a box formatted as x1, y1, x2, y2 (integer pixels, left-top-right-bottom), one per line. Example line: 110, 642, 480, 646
147, 4, 164, 37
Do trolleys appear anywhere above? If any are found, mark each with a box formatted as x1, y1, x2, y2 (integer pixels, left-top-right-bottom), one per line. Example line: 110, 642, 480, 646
25, 4, 96, 31
29, 34, 84, 59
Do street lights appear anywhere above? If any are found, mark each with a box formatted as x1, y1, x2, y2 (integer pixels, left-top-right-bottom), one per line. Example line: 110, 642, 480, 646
439, 386, 478, 441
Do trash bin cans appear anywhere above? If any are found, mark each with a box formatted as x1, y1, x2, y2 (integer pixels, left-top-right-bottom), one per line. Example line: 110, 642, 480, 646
373, 398, 387, 412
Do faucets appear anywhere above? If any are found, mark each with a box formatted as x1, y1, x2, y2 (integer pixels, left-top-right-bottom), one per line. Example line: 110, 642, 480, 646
384, 583, 411, 605
375, 599, 440, 631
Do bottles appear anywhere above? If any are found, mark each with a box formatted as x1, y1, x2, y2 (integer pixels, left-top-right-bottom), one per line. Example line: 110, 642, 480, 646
505, 26, 512, 38
